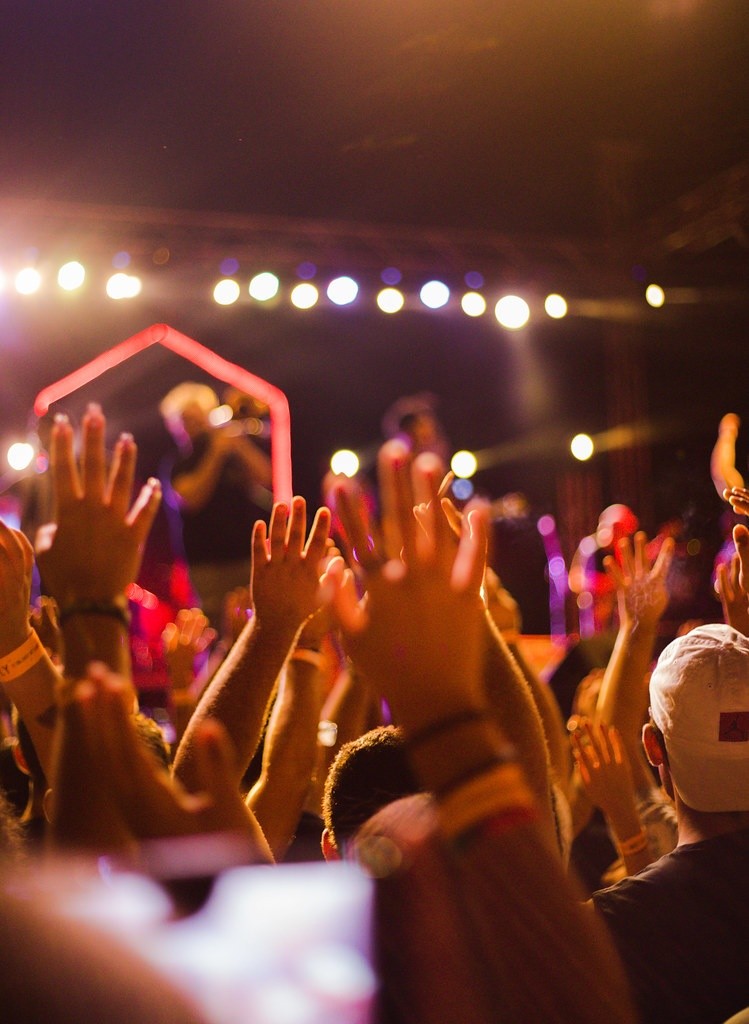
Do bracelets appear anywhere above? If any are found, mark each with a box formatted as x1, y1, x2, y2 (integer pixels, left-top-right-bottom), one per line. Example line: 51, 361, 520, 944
433, 753, 534, 841
618, 825, 650, 857
57, 603, 132, 630
401, 706, 503, 761
0, 627, 45, 684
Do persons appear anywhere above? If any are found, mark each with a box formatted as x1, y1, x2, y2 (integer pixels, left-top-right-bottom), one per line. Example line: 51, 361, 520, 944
0, 382, 748, 1023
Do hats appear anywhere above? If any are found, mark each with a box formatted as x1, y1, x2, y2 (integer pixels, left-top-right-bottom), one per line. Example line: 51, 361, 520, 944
649, 623, 749, 813
